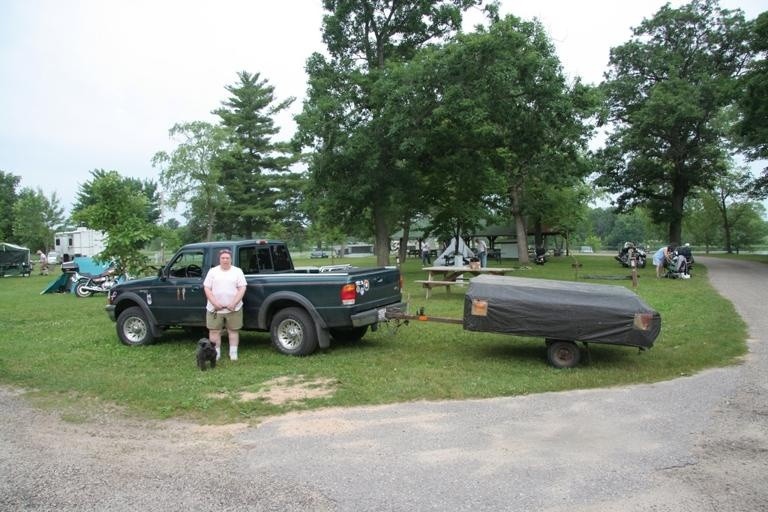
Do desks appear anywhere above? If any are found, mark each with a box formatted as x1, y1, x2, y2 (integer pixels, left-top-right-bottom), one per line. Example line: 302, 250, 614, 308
411, 265, 513, 300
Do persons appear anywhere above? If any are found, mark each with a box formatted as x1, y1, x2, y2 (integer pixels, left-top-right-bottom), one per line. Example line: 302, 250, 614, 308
475, 238, 487, 268
422, 239, 432, 266
652, 244, 675, 277
36, 249, 49, 275
415, 237, 421, 256
203, 249, 248, 362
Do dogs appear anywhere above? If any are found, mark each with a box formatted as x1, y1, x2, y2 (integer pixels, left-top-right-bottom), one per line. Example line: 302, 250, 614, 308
196, 337, 217, 371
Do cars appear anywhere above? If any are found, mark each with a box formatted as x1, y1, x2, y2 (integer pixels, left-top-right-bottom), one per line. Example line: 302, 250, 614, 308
47, 252, 57, 265
579, 246, 593, 253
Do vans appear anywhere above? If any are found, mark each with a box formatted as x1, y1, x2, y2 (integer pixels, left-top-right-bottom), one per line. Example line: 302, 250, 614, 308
310, 246, 336, 258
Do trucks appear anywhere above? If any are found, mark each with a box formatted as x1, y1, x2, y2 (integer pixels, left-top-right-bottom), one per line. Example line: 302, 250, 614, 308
337, 242, 374, 258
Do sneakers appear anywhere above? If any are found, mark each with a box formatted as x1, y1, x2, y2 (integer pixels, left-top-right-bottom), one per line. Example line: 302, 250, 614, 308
216, 352, 239, 362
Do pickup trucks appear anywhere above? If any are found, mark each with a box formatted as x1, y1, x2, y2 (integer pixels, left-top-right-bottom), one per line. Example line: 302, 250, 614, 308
104, 239, 408, 357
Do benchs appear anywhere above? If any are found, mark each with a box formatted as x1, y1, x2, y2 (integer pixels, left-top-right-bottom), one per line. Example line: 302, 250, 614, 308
408, 250, 419, 258
427, 250, 437, 256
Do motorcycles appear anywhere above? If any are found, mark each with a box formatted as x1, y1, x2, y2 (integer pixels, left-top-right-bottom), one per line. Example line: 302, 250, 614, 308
534, 255, 547, 265
61, 259, 135, 298
